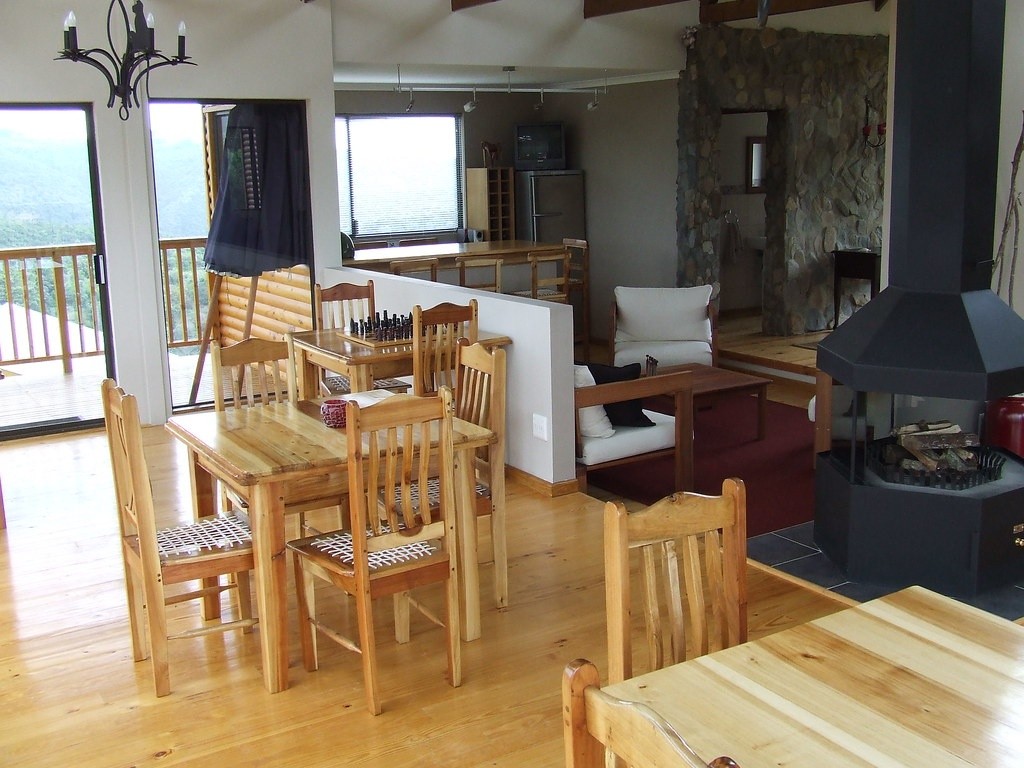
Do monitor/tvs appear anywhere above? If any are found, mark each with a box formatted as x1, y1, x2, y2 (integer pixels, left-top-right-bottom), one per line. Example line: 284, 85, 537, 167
514, 121, 565, 170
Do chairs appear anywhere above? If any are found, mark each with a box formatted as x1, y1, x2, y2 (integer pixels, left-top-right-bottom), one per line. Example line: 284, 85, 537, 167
608, 300, 720, 413
560, 657, 740, 768
353, 240, 389, 250
388, 257, 439, 282
562, 237, 591, 362
100, 279, 510, 717
454, 255, 505, 293
398, 237, 438, 247
507, 249, 572, 304
603, 477, 748, 686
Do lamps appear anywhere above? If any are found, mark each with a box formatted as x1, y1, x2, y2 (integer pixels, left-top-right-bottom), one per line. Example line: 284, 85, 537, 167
463, 100, 479, 113
405, 87, 415, 112
587, 102, 599, 112
535, 88, 546, 110
757, 0, 769, 28
52, 0, 198, 120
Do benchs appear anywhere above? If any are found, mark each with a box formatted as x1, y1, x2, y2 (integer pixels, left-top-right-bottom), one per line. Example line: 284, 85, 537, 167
574, 370, 697, 494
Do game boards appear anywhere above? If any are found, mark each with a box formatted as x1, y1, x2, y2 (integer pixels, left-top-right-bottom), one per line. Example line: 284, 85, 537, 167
335, 319, 460, 347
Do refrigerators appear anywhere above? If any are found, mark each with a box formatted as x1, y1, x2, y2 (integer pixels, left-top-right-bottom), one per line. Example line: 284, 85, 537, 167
514, 170, 585, 332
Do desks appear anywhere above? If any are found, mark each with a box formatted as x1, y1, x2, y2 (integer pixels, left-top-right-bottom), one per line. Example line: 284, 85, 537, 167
342, 239, 565, 275
828, 246, 881, 330
282, 326, 514, 401
641, 362, 773, 442
600, 586, 1024, 768
162, 393, 498, 695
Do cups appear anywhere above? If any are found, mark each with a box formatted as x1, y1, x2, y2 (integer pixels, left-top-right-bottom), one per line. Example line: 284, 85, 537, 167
321, 400, 347, 428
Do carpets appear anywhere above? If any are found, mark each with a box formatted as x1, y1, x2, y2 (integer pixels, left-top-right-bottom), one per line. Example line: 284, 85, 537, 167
591, 396, 815, 539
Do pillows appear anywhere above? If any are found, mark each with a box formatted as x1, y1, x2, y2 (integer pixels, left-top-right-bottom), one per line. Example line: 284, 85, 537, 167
573, 361, 656, 428
614, 283, 712, 344
574, 365, 617, 439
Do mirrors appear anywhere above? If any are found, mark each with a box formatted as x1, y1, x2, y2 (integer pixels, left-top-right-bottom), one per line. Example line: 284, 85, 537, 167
745, 136, 766, 193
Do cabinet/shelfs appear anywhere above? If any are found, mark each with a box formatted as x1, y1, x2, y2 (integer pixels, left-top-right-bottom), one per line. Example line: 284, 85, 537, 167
466, 167, 515, 241
